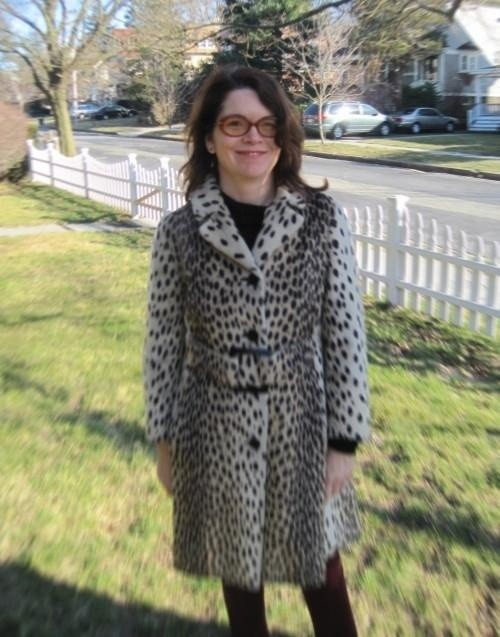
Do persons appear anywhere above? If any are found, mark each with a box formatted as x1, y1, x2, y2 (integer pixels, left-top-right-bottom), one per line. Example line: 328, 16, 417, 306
141, 63, 373, 636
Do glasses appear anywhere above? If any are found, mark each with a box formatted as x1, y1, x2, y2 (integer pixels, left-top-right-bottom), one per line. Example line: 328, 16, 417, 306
211, 114, 280, 137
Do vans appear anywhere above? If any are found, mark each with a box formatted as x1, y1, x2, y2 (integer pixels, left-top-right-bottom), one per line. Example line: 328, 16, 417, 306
301, 101, 396, 140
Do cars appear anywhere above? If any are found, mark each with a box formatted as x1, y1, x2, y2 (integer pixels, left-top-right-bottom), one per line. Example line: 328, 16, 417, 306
24, 97, 139, 120
391, 107, 461, 134
